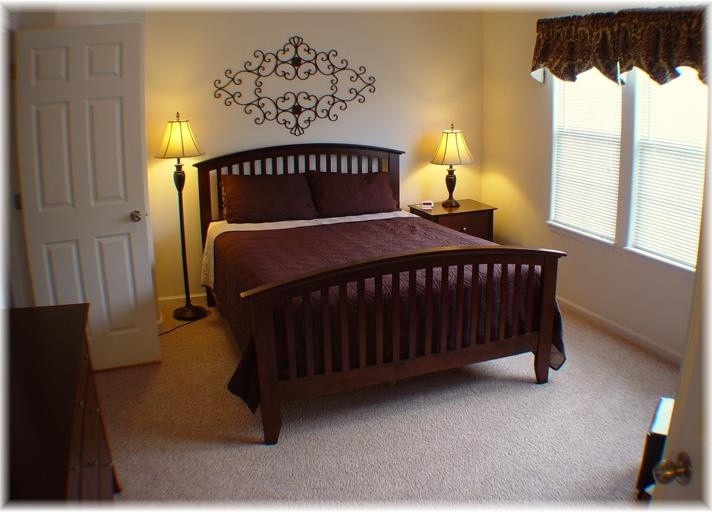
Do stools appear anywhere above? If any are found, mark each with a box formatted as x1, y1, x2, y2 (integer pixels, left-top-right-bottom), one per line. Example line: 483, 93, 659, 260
633, 396, 678, 502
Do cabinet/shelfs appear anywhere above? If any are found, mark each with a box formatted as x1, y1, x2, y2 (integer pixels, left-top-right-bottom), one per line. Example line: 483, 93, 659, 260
4, 302, 124, 500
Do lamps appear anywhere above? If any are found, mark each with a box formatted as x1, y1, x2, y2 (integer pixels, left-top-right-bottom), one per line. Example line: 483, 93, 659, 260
153, 112, 207, 320
431, 122, 476, 209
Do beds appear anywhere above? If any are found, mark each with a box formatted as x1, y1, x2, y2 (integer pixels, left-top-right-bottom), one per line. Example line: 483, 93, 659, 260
186, 145, 571, 445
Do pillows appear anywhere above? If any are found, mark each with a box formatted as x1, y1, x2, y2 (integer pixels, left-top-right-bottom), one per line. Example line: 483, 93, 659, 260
220, 171, 318, 224
306, 169, 401, 218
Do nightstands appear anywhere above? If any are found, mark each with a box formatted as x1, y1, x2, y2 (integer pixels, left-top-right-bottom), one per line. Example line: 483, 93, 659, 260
409, 197, 497, 242
